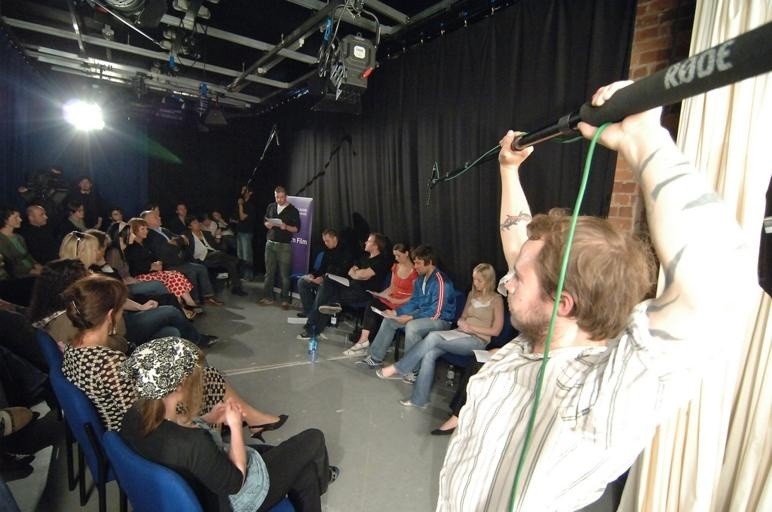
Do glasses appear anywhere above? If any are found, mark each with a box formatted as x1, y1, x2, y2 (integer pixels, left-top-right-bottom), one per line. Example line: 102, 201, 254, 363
74, 231, 84, 258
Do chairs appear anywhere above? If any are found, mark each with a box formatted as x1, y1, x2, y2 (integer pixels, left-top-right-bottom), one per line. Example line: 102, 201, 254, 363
395, 290, 466, 362
47, 360, 116, 508
99, 429, 296, 512
33, 326, 64, 367
289, 251, 326, 305
442, 352, 475, 376
335, 270, 392, 330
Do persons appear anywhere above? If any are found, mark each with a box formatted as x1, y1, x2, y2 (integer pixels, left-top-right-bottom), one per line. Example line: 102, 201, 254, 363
56, 199, 94, 242
165, 201, 190, 234
127, 217, 206, 322
0, 295, 51, 390
377, 262, 505, 409
141, 211, 225, 306
119, 338, 339, 512
296, 226, 358, 318
65, 176, 106, 230
230, 185, 258, 282
85, 229, 170, 294
31, 260, 219, 354
355, 243, 456, 380
149, 204, 179, 242
0, 379, 64, 466
103, 207, 127, 228
212, 210, 237, 256
436, 79, 740, 512
258, 188, 300, 309
106, 221, 196, 319
296, 232, 389, 342
0, 206, 45, 278
343, 241, 420, 356
57, 231, 220, 353
59, 273, 291, 444
431, 348, 507, 435
180, 216, 246, 295
200, 214, 226, 251
23, 203, 60, 263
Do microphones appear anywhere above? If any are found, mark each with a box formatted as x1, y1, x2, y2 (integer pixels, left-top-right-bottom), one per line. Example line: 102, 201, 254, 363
426, 184, 433, 208
273, 124, 280, 145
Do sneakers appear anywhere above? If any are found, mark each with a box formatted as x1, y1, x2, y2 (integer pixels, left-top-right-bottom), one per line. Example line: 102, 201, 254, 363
296, 331, 311, 339
281, 302, 289, 308
354, 355, 384, 370
402, 373, 418, 385
257, 298, 274, 305
240, 277, 252, 282
319, 303, 342, 314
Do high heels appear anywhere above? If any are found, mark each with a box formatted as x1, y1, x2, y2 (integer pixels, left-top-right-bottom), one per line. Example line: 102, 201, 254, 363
184, 305, 204, 315
248, 415, 288, 443
222, 421, 248, 432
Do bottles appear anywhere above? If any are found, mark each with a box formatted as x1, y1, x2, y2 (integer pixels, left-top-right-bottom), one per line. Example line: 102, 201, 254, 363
309, 324, 317, 363
446, 365, 454, 388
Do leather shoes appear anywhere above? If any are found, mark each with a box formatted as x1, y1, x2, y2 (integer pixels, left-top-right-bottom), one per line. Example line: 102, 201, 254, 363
231, 287, 247, 296
198, 335, 219, 349
0, 407, 32, 437
329, 466, 339, 483
203, 298, 224, 307
431, 428, 458, 436
399, 399, 429, 410
1, 454, 35, 468
342, 348, 365, 355
376, 368, 394, 380
350, 340, 370, 350
185, 311, 198, 319
